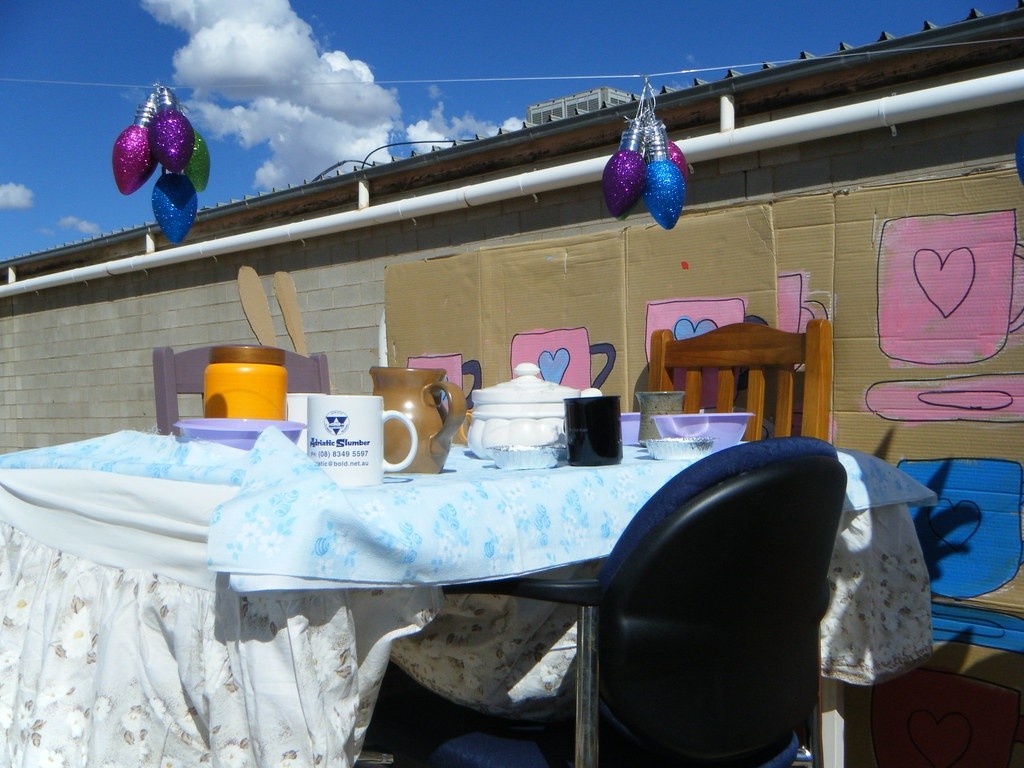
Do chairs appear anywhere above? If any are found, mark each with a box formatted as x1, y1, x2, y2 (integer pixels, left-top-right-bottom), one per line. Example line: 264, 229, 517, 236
431, 437, 854, 767
643, 318, 832, 448
153, 342, 331, 441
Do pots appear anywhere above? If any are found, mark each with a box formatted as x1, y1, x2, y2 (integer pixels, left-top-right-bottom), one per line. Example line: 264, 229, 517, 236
466, 365, 602, 460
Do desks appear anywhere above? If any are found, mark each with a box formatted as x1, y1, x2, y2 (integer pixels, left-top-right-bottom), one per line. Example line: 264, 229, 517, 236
2, 372, 930, 766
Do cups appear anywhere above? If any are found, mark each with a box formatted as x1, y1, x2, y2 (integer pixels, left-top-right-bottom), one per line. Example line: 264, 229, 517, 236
562, 394, 623, 467
635, 390, 685, 448
307, 394, 419, 488
285, 392, 308, 454
369, 366, 467, 475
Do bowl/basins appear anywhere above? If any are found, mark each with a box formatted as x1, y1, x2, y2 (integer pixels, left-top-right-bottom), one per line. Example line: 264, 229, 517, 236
639, 438, 719, 460
485, 444, 567, 469
620, 412, 641, 446
649, 413, 756, 452
172, 417, 306, 451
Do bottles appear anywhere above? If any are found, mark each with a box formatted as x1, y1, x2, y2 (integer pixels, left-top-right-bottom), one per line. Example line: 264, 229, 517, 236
203, 344, 288, 421
